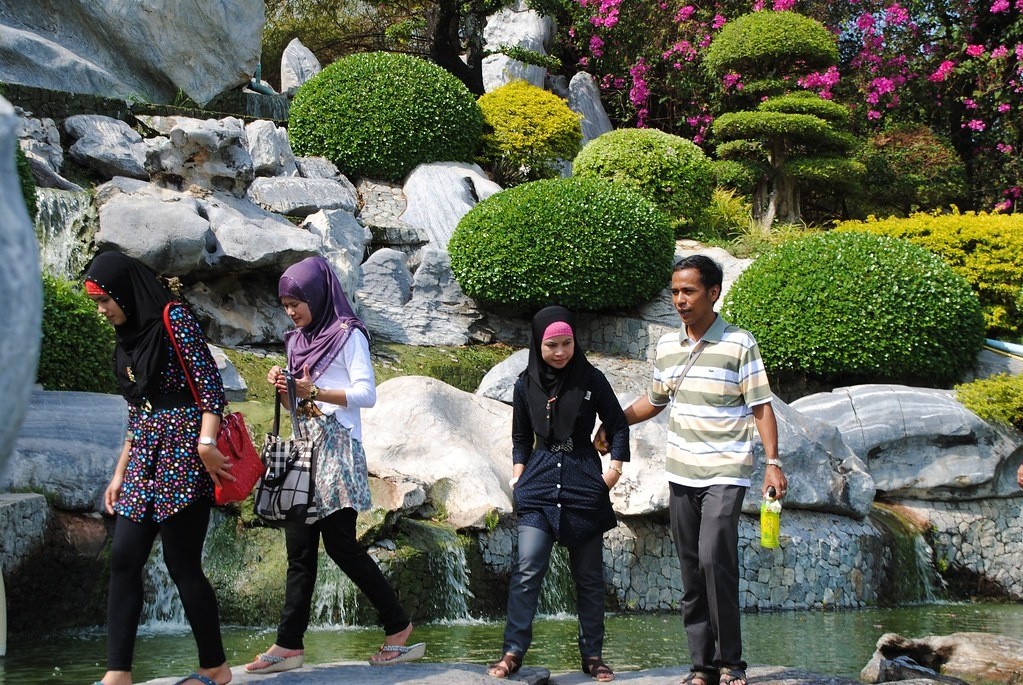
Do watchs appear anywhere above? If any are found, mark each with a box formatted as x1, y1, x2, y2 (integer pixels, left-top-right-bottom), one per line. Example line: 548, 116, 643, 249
197, 437, 217, 446
766, 457, 783, 468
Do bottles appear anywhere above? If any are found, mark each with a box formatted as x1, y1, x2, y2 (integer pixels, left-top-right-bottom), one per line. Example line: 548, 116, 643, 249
760, 487, 781, 550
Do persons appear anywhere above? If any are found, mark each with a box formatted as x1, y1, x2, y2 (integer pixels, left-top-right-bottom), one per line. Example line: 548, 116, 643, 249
592, 255, 786, 685
481, 302, 634, 679
84, 249, 240, 685
241, 255, 424, 673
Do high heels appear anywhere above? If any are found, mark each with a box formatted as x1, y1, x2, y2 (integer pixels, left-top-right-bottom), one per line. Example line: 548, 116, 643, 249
245, 652, 304, 674
372, 641, 428, 665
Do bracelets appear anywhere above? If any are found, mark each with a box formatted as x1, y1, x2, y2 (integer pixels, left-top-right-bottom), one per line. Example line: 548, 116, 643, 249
609, 464, 624, 476
309, 384, 320, 401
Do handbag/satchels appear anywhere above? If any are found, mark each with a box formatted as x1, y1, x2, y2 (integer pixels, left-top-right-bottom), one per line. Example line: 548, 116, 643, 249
212, 409, 265, 504
253, 432, 319, 522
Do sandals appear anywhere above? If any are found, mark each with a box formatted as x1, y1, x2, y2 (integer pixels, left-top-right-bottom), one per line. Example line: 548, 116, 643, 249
582, 659, 612, 681
490, 655, 524, 677
682, 665, 748, 685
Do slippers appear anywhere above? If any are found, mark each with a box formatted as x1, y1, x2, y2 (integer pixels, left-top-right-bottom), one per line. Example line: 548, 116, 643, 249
170, 673, 218, 684
